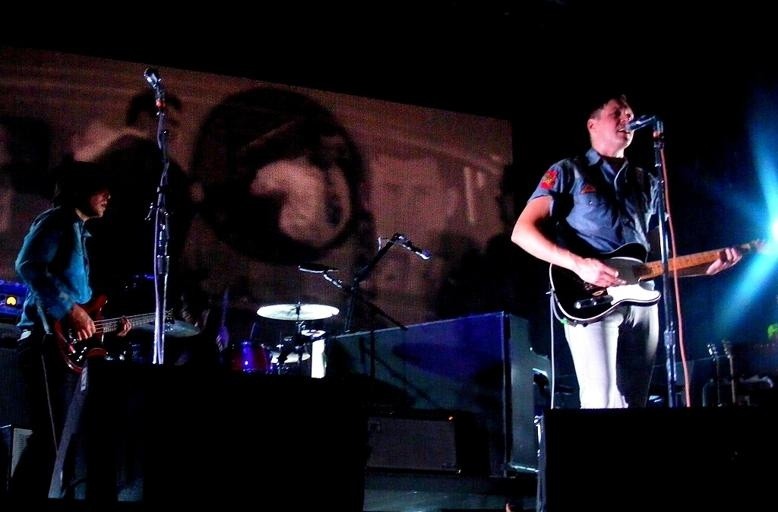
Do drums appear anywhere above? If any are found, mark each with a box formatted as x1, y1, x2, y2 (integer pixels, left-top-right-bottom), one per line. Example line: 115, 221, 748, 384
270, 350, 309, 375
226, 339, 272, 377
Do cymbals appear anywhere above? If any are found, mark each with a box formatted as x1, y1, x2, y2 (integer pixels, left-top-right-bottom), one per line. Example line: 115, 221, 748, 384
257, 304, 336, 320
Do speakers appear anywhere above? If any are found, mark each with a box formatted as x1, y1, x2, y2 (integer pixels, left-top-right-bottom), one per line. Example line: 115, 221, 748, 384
537, 407, 778, 512
364, 407, 473, 479
47, 357, 363, 505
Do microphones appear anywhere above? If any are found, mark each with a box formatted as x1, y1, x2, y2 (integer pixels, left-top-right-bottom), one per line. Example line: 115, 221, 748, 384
400, 241, 432, 260
721, 337, 732, 361
143, 67, 162, 91
706, 343, 720, 365
624, 116, 657, 132
296, 261, 340, 275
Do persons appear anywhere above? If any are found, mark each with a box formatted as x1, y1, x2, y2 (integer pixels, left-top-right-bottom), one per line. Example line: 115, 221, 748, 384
361, 128, 462, 319
13, 160, 111, 499
249, 123, 352, 256
510, 87, 744, 408
43, 85, 194, 501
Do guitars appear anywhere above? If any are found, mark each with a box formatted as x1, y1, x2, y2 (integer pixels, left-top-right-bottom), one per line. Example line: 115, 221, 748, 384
49, 295, 175, 377
548, 238, 764, 327
